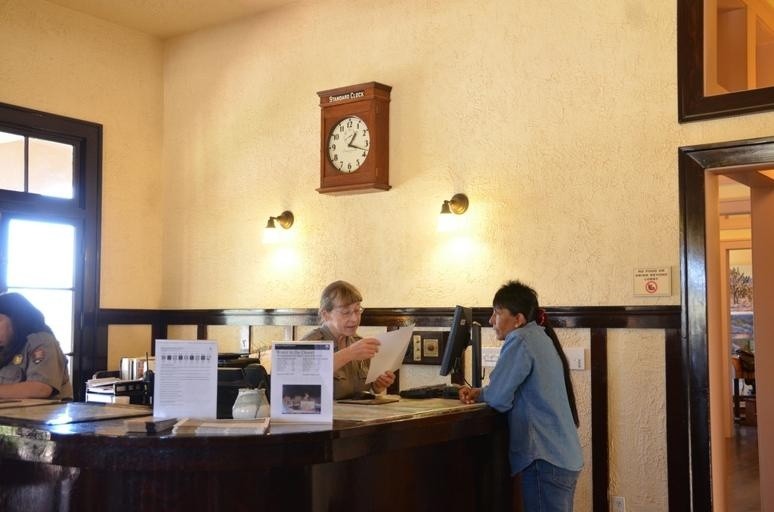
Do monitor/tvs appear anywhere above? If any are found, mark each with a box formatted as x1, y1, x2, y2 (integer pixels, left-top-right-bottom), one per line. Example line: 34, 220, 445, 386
440, 305, 471, 376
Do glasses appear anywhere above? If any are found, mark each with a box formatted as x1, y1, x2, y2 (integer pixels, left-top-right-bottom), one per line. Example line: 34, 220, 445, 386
336, 307, 364, 316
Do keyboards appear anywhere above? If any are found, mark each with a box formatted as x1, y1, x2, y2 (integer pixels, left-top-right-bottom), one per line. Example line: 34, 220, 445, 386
400, 383, 446, 399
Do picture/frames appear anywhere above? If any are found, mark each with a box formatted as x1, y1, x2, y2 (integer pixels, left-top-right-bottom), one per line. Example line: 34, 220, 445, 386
677, 0, 774, 123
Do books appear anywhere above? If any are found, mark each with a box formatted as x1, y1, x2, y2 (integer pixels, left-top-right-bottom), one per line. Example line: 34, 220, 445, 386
86, 356, 156, 393
125, 415, 175, 432
169, 417, 271, 435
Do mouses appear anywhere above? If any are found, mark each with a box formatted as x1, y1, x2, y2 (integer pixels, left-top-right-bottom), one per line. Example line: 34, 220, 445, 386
352, 390, 375, 400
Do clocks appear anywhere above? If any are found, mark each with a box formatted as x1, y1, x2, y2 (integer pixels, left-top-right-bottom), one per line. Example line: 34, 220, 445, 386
316, 82, 393, 196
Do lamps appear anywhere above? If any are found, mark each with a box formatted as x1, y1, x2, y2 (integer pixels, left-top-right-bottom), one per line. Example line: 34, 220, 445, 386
262, 210, 294, 246
437, 193, 468, 234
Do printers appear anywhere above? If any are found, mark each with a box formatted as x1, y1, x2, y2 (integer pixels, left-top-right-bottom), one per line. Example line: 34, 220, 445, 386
217, 352, 270, 419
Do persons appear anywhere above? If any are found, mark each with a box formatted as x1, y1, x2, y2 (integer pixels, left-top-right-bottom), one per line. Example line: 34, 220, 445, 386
0, 291, 74, 403
460, 279, 584, 512
298, 279, 396, 400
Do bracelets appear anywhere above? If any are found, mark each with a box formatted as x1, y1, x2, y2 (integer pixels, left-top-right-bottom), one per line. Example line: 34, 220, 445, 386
369, 386, 387, 397
